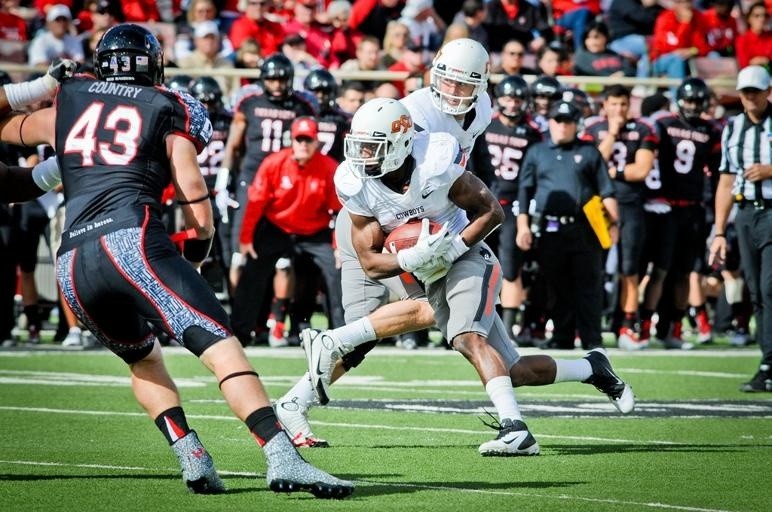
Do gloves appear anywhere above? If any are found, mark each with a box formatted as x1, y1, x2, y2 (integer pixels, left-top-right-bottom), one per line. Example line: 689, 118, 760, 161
213, 189, 239, 223
46, 57, 75, 93
398, 218, 470, 286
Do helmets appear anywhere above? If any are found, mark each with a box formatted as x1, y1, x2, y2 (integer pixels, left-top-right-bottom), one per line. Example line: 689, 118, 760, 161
679, 78, 707, 121
259, 54, 297, 104
428, 38, 491, 116
170, 75, 221, 110
494, 76, 587, 122
94, 23, 163, 87
344, 97, 414, 178
304, 70, 336, 112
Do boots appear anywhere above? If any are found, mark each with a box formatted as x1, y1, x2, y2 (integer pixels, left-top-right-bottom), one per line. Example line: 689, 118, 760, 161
173, 431, 225, 492
262, 431, 354, 498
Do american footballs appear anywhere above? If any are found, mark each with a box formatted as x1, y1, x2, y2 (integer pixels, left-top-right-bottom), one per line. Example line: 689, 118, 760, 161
383, 220, 449, 253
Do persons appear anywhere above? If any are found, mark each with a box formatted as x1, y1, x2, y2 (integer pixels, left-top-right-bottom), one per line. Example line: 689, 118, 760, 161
333, 96, 635, 455
272, 37, 494, 446
0, 1, 772, 392
2, 23, 354, 499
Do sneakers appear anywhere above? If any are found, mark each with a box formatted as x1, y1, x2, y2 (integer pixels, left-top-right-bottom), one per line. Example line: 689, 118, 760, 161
479, 411, 541, 457
513, 321, 750, 350
264, 319, 288, 347
270, 400, 331, 448
582, 347, 635, 415
299, 329, 354, 405
743, 359, 771, 391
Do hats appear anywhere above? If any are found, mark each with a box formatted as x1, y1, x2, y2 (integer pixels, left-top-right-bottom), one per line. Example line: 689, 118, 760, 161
48, 5, 70, 22
290, 117, 318, 140
737, 66, 772, 91
196, 21, 219, 38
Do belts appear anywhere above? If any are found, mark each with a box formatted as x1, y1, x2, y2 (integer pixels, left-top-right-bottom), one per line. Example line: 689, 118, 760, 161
747, 198, 771, 210
